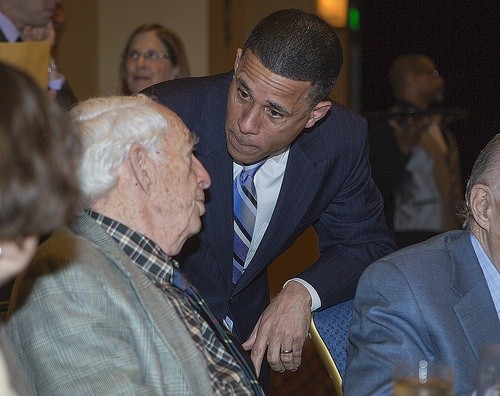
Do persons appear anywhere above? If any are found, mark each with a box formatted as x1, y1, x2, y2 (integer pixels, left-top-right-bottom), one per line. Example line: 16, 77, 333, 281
368, 54, 460, 250
119, 24, 191, 95
340, 133, 500, 396
2, 93, 268, 396
0, 62, 81, 396
0, 0, 78, 110
138, 9, 397, 379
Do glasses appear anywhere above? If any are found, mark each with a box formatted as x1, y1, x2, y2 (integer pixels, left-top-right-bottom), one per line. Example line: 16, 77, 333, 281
125, 49, 170, 62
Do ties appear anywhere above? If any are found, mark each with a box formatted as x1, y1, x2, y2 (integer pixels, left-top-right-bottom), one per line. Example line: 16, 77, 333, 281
233, 155, 272, 286
173, 266, 265, 396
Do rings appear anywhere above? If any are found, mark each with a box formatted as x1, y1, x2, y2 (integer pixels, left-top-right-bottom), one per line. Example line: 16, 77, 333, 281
281, 350, 293, 354
